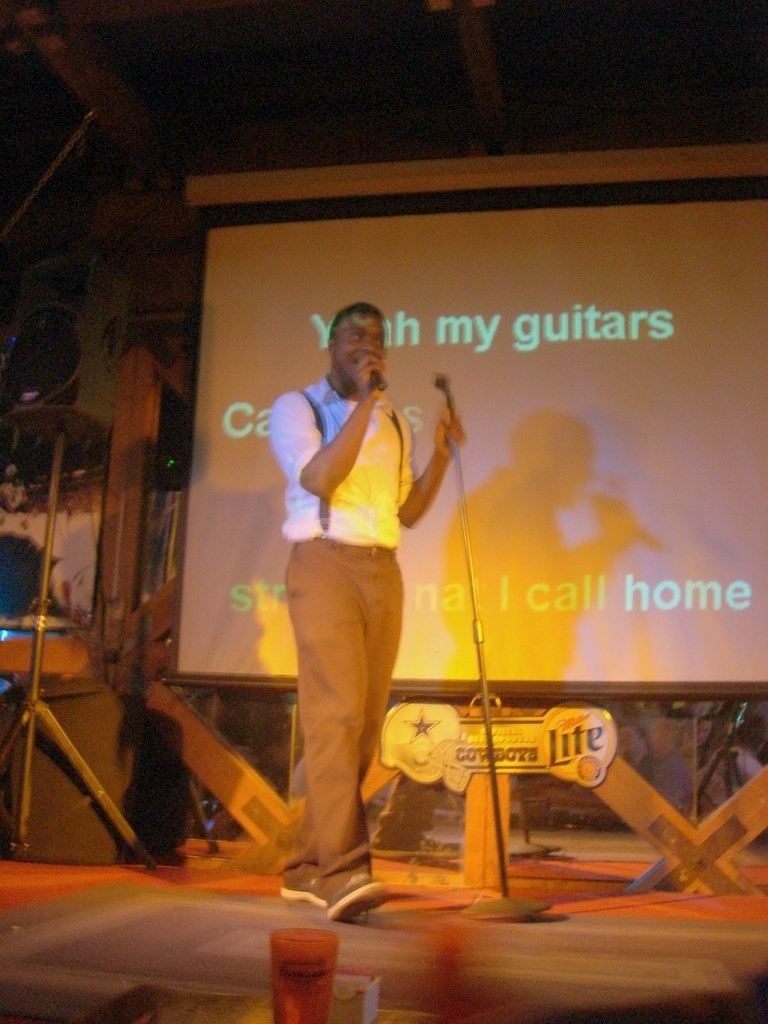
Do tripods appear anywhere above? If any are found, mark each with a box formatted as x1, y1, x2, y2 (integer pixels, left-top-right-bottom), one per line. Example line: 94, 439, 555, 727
0, 432, 161, 874
697, 701, 744, 804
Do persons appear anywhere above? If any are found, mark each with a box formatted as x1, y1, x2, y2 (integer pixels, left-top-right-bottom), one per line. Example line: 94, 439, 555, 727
269, 303, 465, 920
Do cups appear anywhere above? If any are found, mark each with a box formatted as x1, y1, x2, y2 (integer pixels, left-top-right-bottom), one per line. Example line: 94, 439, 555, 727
270, 929, 339, 1024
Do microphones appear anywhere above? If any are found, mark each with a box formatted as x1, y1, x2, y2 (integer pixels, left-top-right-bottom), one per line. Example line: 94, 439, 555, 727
371, 370, 387, 392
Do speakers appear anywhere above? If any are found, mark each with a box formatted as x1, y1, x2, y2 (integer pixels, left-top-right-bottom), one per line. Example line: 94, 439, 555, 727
0, 252, 131, 445
0, 684, 148, 866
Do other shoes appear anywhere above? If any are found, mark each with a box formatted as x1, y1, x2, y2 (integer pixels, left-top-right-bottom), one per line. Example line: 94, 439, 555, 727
280, 863, 328, 908
328, 871, 392, 922
